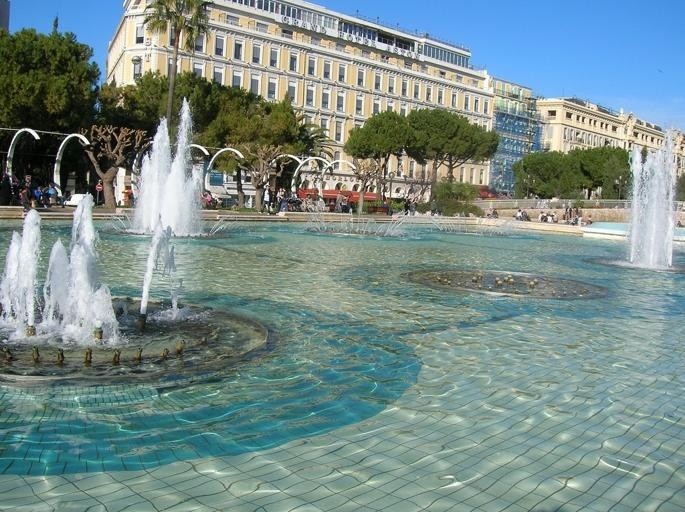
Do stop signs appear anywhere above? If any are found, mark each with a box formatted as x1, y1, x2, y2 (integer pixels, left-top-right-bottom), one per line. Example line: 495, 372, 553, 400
94, 184, 102, 191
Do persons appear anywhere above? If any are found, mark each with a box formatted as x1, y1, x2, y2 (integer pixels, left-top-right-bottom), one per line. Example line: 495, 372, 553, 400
431, 199, 437, 216
257, 185, 321, 216
488, 204, 593, 225
20, 183, 66, 209
200, 191, 212, 209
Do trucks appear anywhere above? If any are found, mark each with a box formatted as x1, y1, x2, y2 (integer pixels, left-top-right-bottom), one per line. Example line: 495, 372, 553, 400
61, 193, 86, 207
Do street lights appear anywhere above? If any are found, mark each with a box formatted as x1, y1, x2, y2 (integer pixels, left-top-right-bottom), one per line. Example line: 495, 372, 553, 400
387, 171, 396, 198
132, 55, 143, 85
615, 175, 628, 199
523, 174, 536, 197
